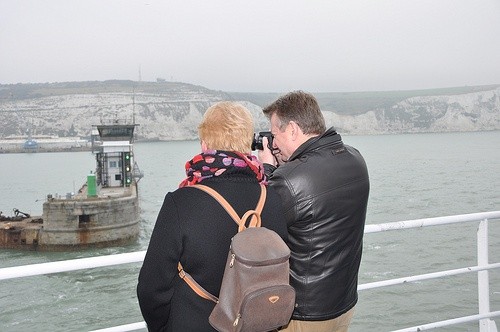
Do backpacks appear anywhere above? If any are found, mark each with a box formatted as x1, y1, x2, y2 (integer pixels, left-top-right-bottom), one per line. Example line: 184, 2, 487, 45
176, 176, 297, 332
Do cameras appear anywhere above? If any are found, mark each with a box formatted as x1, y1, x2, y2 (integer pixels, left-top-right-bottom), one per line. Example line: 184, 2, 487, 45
252, 131, 273, 151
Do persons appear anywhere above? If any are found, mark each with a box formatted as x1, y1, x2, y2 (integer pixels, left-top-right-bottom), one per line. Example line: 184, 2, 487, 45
255, 88, 371, 332
134, 101, 296, 332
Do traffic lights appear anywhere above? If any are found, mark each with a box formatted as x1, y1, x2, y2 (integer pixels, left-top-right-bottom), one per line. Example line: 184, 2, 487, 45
124, 152, 131, 185
